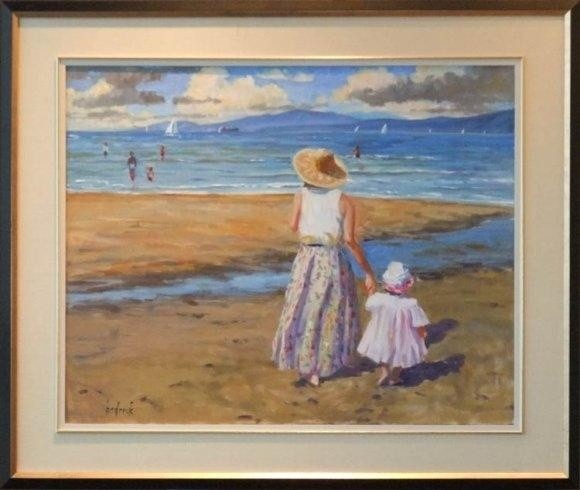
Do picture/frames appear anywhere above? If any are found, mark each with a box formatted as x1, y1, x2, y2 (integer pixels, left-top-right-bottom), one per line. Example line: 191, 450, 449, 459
0, 0, 580, 490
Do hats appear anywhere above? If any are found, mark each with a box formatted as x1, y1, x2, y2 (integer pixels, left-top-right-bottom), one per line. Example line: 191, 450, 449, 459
292, 146, 348, 190
381, 260, 415, 294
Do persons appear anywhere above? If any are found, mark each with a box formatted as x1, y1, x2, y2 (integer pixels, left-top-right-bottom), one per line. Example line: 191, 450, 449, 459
147, 167, 155, 181
159, 145, 165, 161
126, 151, 137, 181
102, 141, 109, 158
355, 261, 430, 387
352, 145, 360, 158
269, 145, 378, 387
